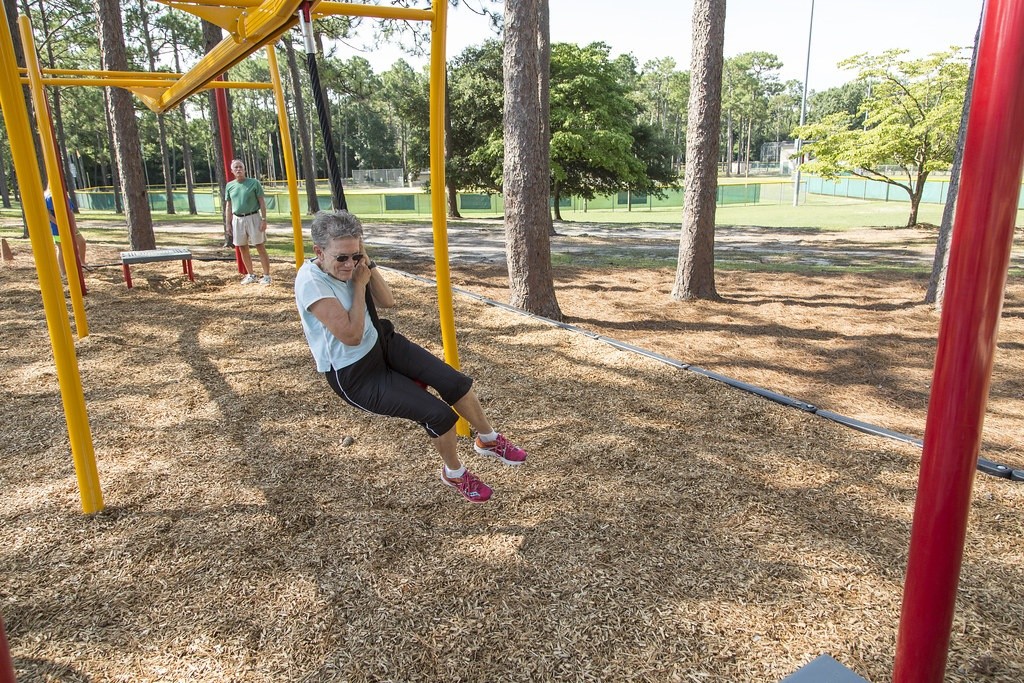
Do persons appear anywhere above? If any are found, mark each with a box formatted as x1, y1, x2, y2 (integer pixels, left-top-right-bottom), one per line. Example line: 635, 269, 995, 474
294, 210, 528, 505
44, 181, 93, 278
225, 161, 271, 285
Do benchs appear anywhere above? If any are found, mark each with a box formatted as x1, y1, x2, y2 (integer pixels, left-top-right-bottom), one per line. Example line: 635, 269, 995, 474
121, 247, 194, 289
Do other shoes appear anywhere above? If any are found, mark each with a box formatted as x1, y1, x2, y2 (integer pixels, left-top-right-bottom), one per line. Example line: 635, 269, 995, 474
81, 263, 94, 272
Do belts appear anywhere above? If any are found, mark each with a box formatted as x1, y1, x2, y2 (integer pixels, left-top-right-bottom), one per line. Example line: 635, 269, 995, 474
234, 210, 258, 217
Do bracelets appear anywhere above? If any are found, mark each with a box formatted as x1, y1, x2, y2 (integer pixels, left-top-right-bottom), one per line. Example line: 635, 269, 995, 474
262, 218, 266, 221
368, 261, 376, 268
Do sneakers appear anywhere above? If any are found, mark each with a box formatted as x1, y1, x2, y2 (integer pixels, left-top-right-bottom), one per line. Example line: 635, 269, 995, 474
440, 464, 494, 504
260, 275, 272, 284
240, 274, 257, 285
474, 433, 528, 465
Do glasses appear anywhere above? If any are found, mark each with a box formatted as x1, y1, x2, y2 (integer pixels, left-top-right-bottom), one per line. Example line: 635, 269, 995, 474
324, 249, 363, 262
